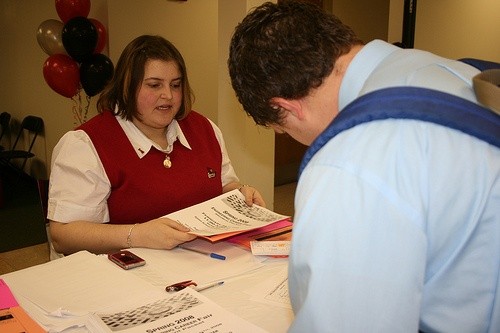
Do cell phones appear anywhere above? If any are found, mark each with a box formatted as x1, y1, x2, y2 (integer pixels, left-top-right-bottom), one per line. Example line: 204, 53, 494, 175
108, 251, 146, 270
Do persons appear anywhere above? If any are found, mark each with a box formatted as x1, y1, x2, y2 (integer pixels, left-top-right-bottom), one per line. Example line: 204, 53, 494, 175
45, 35, 264, 255
226, 0, 500, 333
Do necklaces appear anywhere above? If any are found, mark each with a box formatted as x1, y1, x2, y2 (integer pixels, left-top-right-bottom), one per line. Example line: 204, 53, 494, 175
163, 155, 172, 169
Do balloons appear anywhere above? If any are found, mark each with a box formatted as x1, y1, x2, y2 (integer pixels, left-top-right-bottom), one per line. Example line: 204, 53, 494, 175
36, 0, 113, 98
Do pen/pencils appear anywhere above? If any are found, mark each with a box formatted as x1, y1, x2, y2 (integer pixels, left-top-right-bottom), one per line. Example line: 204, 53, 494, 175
177, 245, 226, 262
193, 281, 224, 292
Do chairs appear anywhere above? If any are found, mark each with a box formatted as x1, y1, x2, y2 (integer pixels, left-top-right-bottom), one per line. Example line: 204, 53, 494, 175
0, 115, 44, 187
0, 112, 11, 167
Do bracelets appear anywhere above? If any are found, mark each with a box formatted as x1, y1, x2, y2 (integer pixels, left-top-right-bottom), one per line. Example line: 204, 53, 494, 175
238, 183, 248, 191
126, 223, 140, 249
